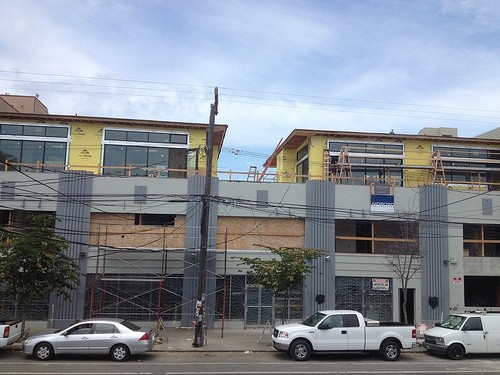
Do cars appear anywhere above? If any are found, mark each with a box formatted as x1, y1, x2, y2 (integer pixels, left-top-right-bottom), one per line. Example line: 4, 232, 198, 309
24, 318, 154, 360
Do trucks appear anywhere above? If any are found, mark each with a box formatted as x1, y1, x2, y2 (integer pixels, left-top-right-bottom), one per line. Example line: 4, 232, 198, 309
422, 311, 500, 359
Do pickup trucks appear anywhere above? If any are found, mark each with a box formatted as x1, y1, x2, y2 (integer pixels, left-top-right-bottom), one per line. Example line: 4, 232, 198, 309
271, 309, 417, 360
0, 318, 22, 352
